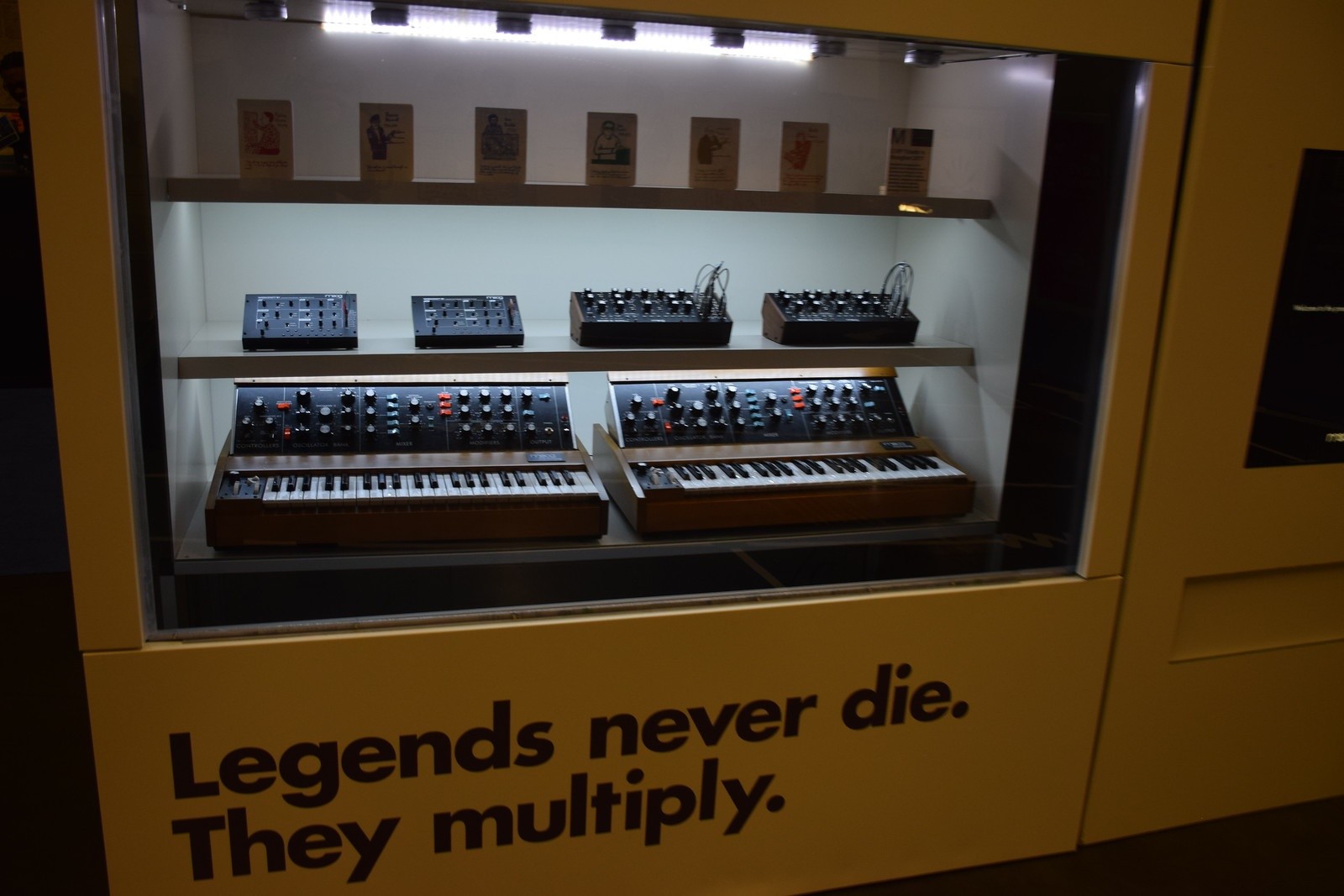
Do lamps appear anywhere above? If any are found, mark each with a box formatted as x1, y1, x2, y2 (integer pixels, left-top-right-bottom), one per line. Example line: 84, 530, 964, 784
599, 24, 636, 42
494, 17, 532, 35
709, 33, 744, 49
903, 53, 939, 69
371, 8, 409, 27
811, 40, 846, 57
243, 0, 288, 21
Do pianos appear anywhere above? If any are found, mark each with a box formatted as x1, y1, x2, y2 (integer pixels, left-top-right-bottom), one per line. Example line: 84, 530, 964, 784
591, 367, 980, 538
201, 373, 611, 546
